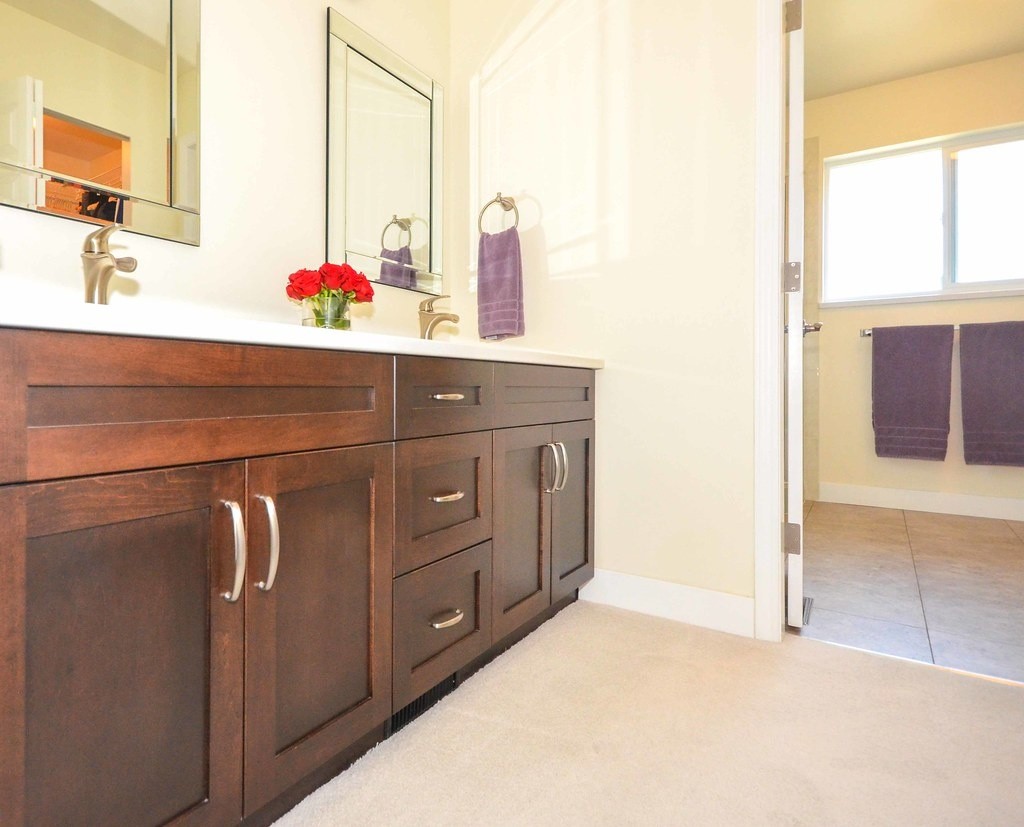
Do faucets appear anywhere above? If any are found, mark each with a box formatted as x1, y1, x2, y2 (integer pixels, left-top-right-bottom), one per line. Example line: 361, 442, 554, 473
81, 223, 137, 304
420, 293, 459, 339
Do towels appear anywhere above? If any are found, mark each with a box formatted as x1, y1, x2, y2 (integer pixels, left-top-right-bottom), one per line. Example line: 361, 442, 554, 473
958, 318, 1024, 469
379, 245, 417, 288
871, 323, 954, 465
476, 226, 526, 343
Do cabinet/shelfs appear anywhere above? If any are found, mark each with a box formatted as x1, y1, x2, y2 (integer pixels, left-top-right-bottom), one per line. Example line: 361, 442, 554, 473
0, 330, 602, 827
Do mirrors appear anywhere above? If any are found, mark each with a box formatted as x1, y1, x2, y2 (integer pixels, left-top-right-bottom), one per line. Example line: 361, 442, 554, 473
0, 0, 201, 248
325, 6, 445, 298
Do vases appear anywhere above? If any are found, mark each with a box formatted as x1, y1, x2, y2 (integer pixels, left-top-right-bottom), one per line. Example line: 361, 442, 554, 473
302, 297, 350, 330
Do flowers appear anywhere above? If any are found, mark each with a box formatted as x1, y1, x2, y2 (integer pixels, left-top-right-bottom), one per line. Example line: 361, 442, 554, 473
286, 262, 374, 326
